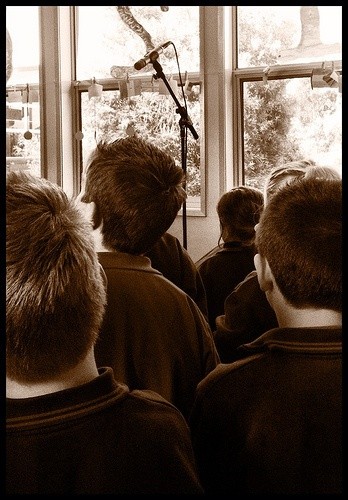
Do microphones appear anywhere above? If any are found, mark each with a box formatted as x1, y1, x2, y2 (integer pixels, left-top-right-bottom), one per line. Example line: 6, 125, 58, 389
135, 41, 172, 71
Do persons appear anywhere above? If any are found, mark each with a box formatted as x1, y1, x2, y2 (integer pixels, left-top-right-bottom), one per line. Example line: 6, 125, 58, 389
6, 172, 206, 493
189, 179, 343, 493
148, 232, 208, 316
82, 137, 220, 419
194, 186, 265, 317
214, 161, 339, 359
